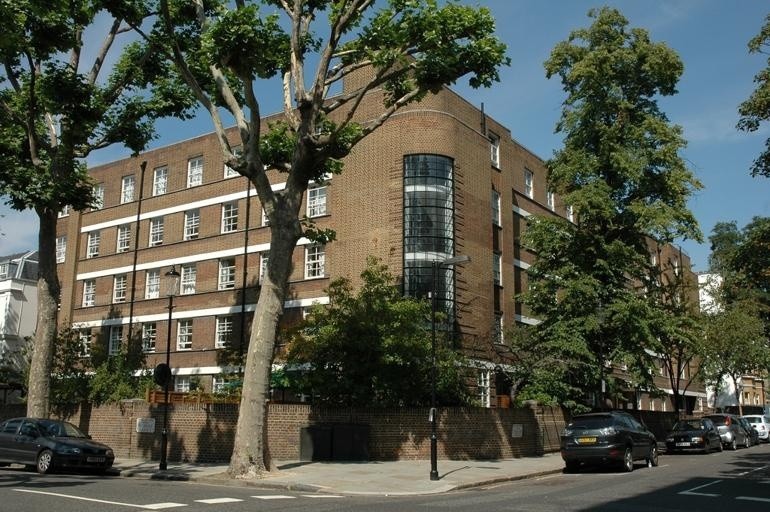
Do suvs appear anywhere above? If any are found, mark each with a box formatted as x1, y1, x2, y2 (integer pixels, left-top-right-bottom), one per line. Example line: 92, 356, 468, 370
560, 412, 658, 471
667, 414, 770, 452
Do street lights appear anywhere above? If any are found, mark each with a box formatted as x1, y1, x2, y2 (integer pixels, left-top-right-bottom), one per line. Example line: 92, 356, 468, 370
159, 265, 180, 470
427, 255, 470, 482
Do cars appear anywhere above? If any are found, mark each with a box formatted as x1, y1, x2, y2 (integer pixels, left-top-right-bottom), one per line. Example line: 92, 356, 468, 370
0, 418, 115, 474
